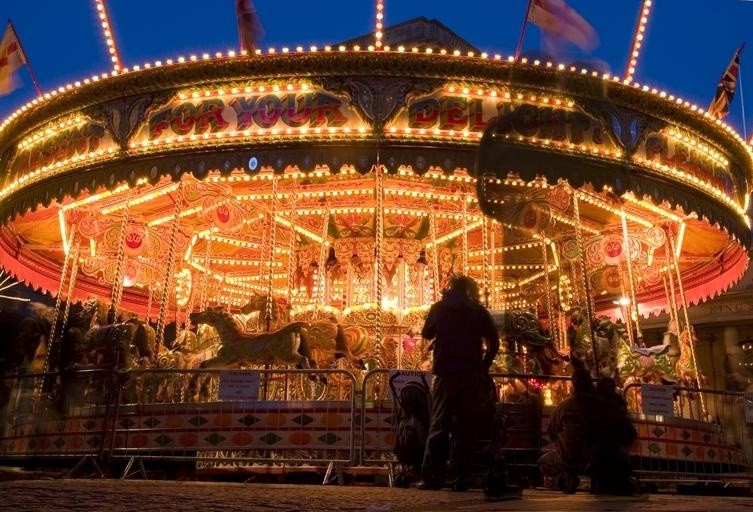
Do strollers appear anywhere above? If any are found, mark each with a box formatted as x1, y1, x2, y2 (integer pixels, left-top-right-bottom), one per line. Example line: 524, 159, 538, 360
387, 371, 465, 491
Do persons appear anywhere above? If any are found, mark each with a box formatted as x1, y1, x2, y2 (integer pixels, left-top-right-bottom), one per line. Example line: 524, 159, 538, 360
409, 273, 500, 490
594, 377, 636, 494
538, 369, 638, 492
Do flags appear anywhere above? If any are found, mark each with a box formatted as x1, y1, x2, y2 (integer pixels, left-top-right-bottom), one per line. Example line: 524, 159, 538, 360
0, 16, 45, 107
518, 0, 600, 64
